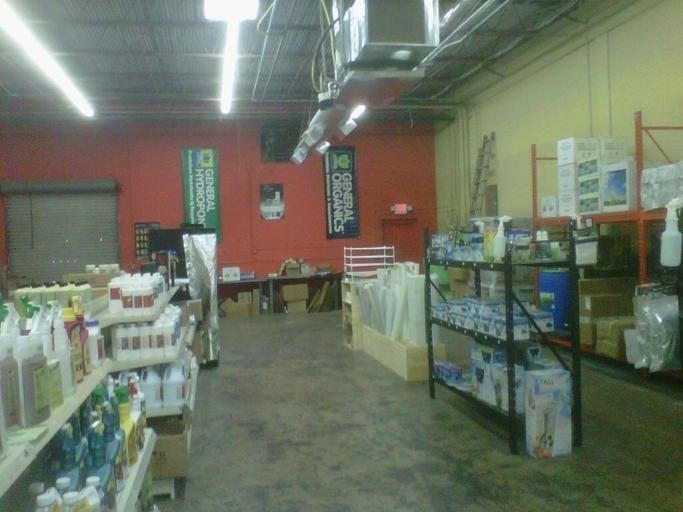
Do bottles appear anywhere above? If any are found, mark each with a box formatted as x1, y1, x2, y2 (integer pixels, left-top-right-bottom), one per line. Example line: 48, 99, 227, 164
535, 230, 548, 241
107, 271, 166, 311
0, 294, 149, 512
108, 302, 182, 360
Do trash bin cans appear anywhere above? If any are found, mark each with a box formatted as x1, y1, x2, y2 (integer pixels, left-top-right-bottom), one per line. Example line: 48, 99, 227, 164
527, 370, 572, 458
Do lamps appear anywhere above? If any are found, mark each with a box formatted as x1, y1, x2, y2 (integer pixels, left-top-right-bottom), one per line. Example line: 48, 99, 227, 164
288, 118, 358, 165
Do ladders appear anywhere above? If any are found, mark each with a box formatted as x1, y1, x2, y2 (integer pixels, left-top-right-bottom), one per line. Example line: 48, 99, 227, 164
469, 132, 495, 217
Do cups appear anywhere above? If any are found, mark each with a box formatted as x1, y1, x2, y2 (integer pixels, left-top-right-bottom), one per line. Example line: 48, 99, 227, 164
534, 241, 551, 258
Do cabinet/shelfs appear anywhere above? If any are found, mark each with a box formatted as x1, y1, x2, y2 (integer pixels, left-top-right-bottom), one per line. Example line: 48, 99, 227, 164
340, 280, 360, 351
528, 109, 681, 382
0, 274, 203, 512
342, 245, 395, 281
422, 225, 581, 457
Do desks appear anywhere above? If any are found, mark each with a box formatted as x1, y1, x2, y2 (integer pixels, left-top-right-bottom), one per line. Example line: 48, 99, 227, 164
265, 274, 338, 313
215, 276, 267, 315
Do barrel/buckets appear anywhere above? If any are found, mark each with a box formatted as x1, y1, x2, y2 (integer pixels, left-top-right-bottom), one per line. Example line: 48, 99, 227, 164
539, 271, 581, 331
116, 363, 185, 406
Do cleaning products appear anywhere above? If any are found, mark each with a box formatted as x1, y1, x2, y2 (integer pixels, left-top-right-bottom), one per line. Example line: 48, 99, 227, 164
659, 197, 682, 269
0, 294, 107, 427
29, 372, 146, 511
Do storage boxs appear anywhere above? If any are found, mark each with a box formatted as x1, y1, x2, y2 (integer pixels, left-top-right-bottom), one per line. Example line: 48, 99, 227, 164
538, 135, 678, 219
218, 289, 260, 317
286, 299, 306, 313
278, 282, 309, 301
575, 275, 635, 363
